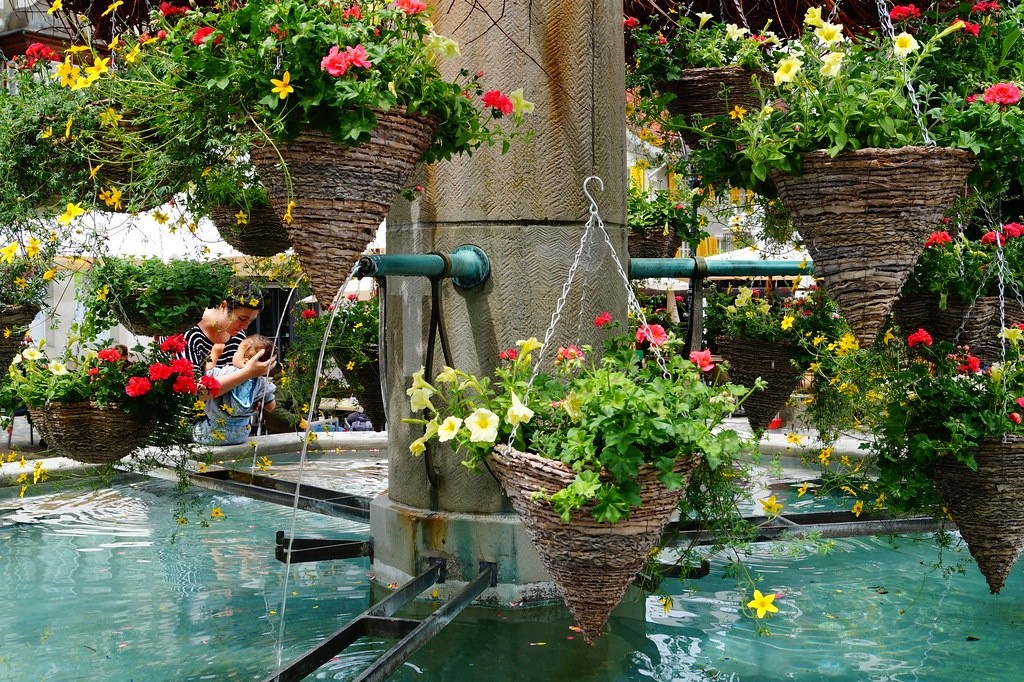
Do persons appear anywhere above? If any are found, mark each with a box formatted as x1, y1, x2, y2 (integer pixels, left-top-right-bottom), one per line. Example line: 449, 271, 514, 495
190, 334, 276, 445
145, 277, 277, 446
265, 384, 321, 435
115, 345, 134, 372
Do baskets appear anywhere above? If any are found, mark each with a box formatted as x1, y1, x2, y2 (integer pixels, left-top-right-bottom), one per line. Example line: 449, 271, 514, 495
654, 76, 705, 150
764, 146, 978, 349
488, 443, 704, 646
929, 434, 1024, 595
894, 292, 1024, 377
628, 226, 682, 258
329, 344, 387, 432
236, 103, 442, 309
38, 98, 201, 214
26, 401, 157, 464
715, 335, 823, 440
0, 304, 41, 383
679, 66, 774, 147
207, 194, 293, 258
112, 289, 205, 336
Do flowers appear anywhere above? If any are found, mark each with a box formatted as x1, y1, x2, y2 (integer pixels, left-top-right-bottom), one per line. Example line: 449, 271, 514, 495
0, 0, 1024, 619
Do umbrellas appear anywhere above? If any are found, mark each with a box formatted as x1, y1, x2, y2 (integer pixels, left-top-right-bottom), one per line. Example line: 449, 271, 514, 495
634, 228, 818, 297
299, 274, 380, 305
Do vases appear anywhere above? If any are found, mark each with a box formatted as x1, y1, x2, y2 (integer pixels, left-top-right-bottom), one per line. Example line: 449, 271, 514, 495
654, 66, 777, 148
894, 292, 937, 348
905, 430, 1024, 597
109, 288, 205, 337
28, 400, 159, 464
626, 224, 682, 259
484, 444, 703, 647
206, 204, 291, 257
328, 345, 388, 433
234, 104, 439, 312
982, 296, 1024, 360
0, 302, 41, 383
713, 335, 810, 440
61, 107, 198, 215
930, 295, 999, 357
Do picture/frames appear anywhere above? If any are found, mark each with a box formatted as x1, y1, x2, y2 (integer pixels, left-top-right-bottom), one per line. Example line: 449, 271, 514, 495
768, 143, 974, 348
